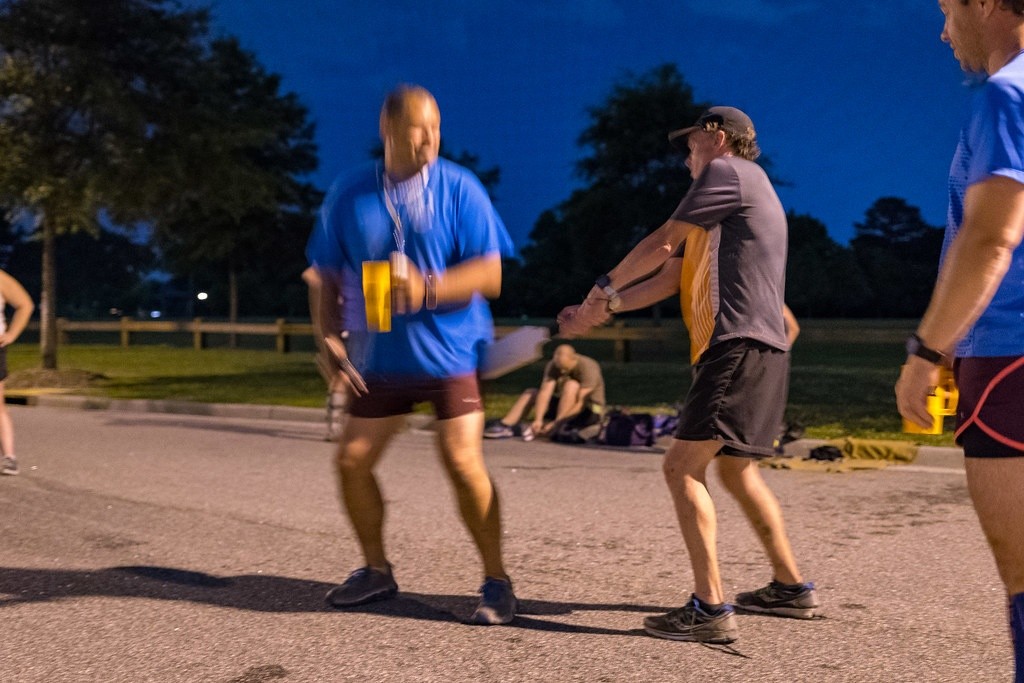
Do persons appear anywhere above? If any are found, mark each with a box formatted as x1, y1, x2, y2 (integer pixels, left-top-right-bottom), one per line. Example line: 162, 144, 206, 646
894, 0, 1024, 683
302, 84, 517, 626
0, 266, 34, 476
557, 106, 822, 642
485, 343, 607, 441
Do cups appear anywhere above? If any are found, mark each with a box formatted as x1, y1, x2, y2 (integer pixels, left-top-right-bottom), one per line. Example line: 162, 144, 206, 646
899, 364, 959, 437
362, 261, 392, 334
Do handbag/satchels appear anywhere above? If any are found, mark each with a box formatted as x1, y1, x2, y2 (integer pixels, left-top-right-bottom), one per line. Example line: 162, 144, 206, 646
551, 409, 679, 447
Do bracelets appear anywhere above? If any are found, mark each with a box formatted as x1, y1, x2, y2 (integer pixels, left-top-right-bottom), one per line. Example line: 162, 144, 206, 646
426, 273, 437, 310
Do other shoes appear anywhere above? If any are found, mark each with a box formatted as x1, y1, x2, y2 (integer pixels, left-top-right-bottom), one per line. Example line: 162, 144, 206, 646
0, 456, 19, 475
483, 420, 514, 438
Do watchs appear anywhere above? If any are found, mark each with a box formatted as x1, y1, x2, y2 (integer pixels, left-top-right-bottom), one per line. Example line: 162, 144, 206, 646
594, 273, 616, 298
905, 333, 942, 364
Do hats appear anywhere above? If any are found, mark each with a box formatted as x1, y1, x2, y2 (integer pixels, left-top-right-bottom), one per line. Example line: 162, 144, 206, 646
667, 106, 756, 152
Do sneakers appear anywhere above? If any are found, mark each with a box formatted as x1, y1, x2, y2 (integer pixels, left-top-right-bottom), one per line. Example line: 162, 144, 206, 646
327, 562, 398, 608
643, 593, 740, 644
470, 574, 516, 624
735, 575, 818, 620
